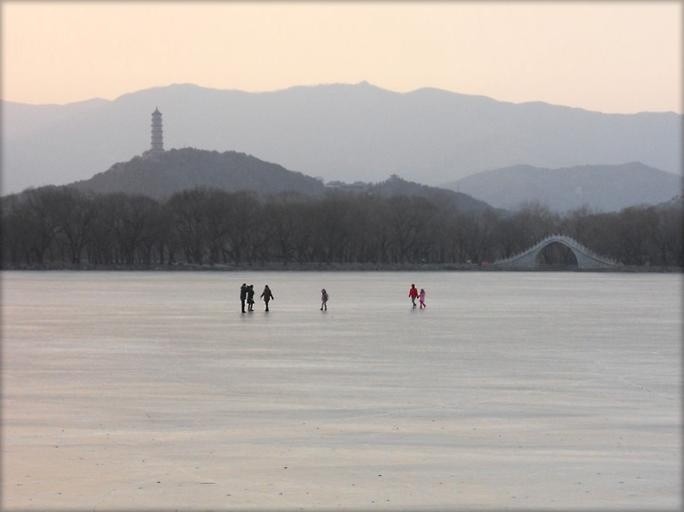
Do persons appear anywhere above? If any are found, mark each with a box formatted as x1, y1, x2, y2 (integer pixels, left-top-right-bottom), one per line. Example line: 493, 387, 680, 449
320, 289, 328, 311
261, 285, 274, 311
240, 283, 254, 313
409, 284, 426, 309
